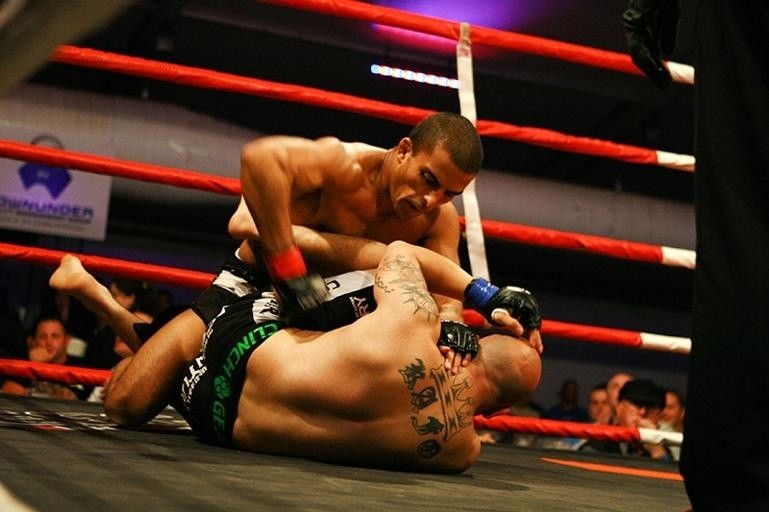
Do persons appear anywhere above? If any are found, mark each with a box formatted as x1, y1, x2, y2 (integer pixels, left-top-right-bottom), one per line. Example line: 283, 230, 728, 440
49, 238, 544, 474
491, 371, 685, 464
0, 274, 175, 400
103, 111, 484, 425
626, 0, 769, 511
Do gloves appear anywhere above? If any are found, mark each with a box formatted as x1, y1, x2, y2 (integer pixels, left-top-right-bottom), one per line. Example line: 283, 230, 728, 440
621, 0, 671, 90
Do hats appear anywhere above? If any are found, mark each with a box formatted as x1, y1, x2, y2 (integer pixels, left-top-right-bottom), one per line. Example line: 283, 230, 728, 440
620, 379, 665, 407
